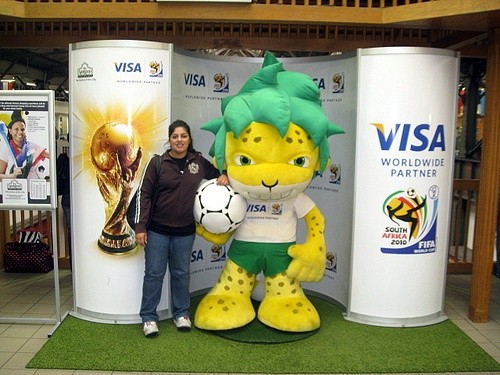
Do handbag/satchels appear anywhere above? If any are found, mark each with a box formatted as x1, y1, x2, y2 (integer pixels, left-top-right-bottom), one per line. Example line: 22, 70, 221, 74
126, 154, 160, 234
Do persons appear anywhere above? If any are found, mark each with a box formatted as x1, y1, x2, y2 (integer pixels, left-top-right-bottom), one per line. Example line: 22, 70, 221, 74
0, 117, 49, 181
133, 119, 229, 337
56, 132, 73, 275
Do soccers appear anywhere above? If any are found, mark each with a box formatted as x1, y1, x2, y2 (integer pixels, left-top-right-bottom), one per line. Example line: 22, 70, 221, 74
191, 176, 248, 236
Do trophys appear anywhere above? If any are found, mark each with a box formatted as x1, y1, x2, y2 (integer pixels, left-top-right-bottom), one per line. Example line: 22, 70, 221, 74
91, 122, 143, 257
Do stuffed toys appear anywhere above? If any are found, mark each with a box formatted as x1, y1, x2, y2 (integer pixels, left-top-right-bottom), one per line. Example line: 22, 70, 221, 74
194, 50, 349, 332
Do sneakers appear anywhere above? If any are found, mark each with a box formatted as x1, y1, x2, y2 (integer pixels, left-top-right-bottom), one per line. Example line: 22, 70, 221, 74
173, 316, 191, 329
143, 321, 159, 337
